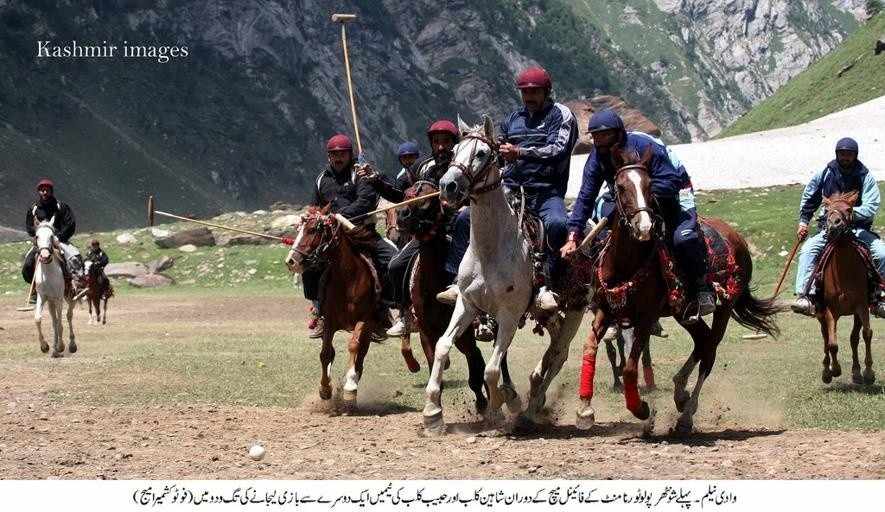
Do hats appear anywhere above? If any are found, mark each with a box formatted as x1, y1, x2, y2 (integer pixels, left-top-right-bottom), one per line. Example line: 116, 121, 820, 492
427, 120, 458, 137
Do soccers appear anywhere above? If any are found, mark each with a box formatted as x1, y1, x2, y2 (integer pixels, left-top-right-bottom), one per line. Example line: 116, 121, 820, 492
250, 446, 265, 462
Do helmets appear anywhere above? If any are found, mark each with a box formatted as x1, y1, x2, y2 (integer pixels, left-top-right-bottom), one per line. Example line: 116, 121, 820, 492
327, 134, 352, 152
586, 110, 624, 135
37, 179, 53, 191
515, 68, 552, 89
91, 240, 99, 244
398, 142, 418, 156
836, 138, 858, 151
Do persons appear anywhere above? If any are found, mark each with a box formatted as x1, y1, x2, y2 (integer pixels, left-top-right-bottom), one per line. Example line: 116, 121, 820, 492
23, 179, 84, 301
387, 120, 495, 341
436, 67, 579, 314
790, 138, 885, 318
591, 183, 669, 341
395, 142, 420, 192
560, 110, 717, 315
82, 240, 108, 300
308, 135, 397, 342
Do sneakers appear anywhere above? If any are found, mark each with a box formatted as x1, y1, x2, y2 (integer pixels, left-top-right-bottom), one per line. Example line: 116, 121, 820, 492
603, 327, 618, 340
386, 309, 420, 336
309, 317, 325, 338
698, 292, 715, 316
29, 295, 37, 303
534, 286, 560, 309
791, 298, 813, 316
72, 288, 90, 301
478, 324, 494, 340
871, 299, 885, 318
651, 321, 668, 337
436, 284, 457, 304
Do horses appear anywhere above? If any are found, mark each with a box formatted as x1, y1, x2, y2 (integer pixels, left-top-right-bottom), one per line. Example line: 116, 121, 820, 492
576, 142, 793, 442
283, 112, 659, 438
31, 213, 111, 358
814, 190, 875, 386
